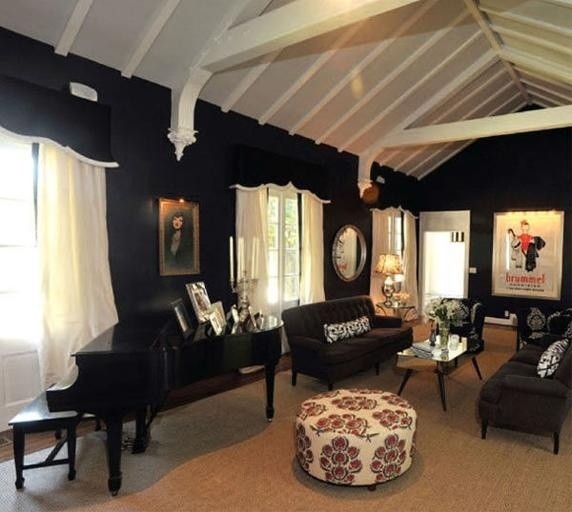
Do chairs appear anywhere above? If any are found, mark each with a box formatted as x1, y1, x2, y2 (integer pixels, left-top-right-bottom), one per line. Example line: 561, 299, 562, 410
515, 305, 572, 352
470, 302, 485, 334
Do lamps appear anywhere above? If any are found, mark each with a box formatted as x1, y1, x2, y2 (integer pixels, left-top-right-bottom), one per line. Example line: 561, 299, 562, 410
373, 255, 404, 315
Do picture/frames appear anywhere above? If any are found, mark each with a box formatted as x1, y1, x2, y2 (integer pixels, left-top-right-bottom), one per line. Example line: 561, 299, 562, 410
491, 210, 564, 301
186, 280, 208, 323
160, 199, 199, 277
215, 307, 226, 325
207, 310, 219, 334
171, 298, 193, 340
213, 302, 225, 327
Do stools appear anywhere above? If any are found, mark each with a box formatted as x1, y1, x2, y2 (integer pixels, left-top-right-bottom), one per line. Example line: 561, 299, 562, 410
294, 388, 418, 491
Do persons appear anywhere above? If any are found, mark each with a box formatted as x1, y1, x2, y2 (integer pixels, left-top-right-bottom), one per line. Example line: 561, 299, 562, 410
169, 210, 191, 270
518, 221, 535, 254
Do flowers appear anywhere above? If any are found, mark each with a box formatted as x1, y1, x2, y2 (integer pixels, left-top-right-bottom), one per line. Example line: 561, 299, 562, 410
423, 295, 468, 328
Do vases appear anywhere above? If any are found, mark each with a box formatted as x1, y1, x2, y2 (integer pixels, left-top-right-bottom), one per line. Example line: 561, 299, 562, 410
439, 321, 448, 348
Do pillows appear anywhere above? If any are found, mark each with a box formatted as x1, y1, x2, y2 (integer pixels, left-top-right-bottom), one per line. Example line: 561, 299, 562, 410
538, 339, 569, 377
322, 315, 372, 342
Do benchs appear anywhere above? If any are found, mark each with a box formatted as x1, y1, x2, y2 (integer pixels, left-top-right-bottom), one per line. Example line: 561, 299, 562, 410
6, 382, 105, 489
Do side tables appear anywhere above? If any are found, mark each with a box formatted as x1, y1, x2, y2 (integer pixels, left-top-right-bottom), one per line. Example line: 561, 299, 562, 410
375, 300, 414, 320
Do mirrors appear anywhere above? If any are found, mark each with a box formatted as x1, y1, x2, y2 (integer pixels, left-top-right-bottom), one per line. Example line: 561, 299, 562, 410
333, 225, 367, 282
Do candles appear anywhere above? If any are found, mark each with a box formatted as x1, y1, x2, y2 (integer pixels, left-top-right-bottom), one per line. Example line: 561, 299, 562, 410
236, 238, 241, 283
228, 235, 235, 292
240, 237, 246, 280
251, 236, 256, 279
255, 238, 260, 280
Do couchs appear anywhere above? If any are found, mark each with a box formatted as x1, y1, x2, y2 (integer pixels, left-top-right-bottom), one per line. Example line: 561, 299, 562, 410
479, 332, 572, 456
280, 295, 414, 391
